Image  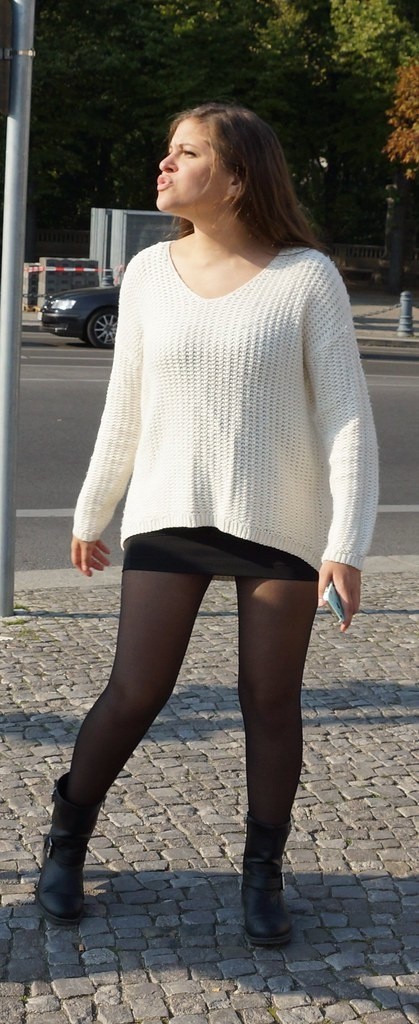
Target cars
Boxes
[37,284,120,350]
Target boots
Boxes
[35,772,107,927]
[240,811,292,944]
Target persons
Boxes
[34,105,380,947]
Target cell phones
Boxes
[323,581,345,623]
